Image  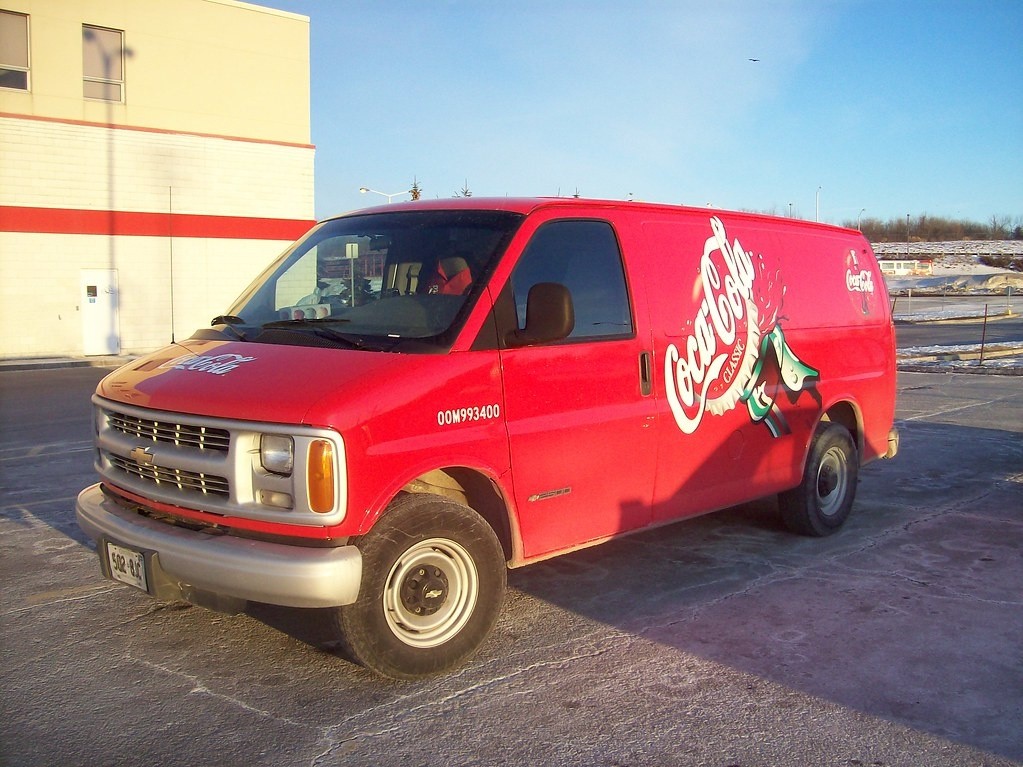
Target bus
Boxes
[876,259,934,276]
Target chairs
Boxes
[423,255,471,295]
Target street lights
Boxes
[815,185,823,222]
[906,213,911,242]
[856,208,867,230]
[788,202,793,218]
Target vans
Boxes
[74,194,900,682]
